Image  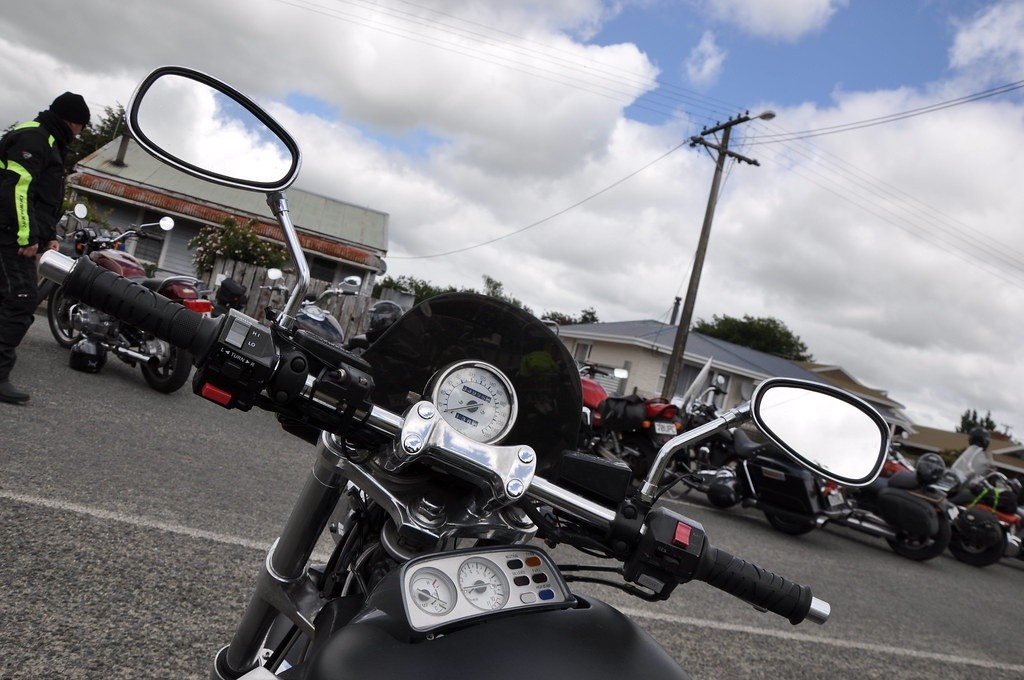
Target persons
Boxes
[0,90,91,402]
[212,277,248,318]
[952,425,995,483]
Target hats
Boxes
[50,92,90,124]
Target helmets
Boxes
[968,426,990,451]
[365,300,404,343]
[70,339,107,373]
[916,453,946,484]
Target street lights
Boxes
[658,109,780,401]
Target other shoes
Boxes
[0,381,30,401]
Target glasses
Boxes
[81,123,85,131]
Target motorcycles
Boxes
[40,59,890,679]
[45,199,1024,575]
